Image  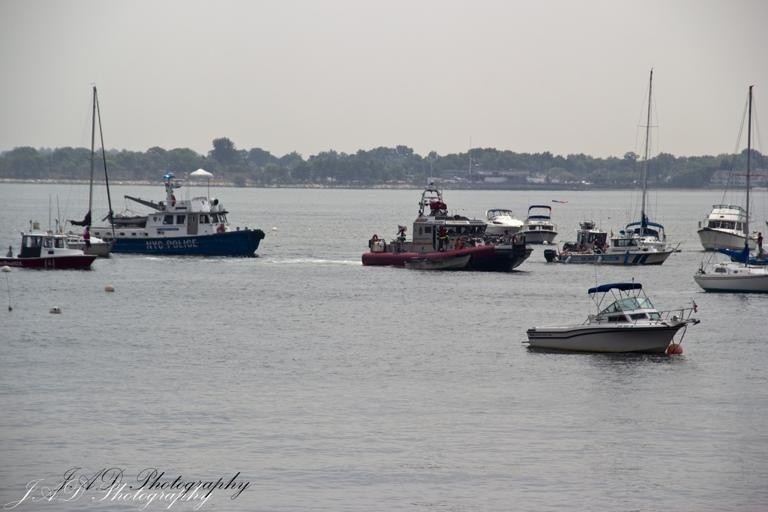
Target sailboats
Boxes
[691,82,768,295]
[626,65,671,242]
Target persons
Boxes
[498,229,512,240]
[83,227,91,249]
[756,232,763,252]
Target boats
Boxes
[0,229,100,269]
[51,83,120,257]
[524,275,706,355]
[692,202,759,251]
[90,165,271,260]
